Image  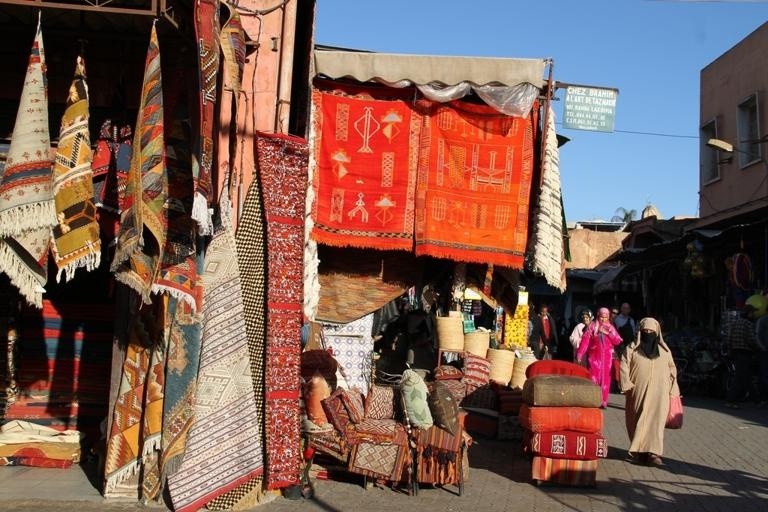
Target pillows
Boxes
[306,370,365,434]
[400,368,460,436]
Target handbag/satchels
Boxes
[663,392,683,430]
[611,358,620,382]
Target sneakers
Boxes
[626,452,667,467]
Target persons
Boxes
[618,316,681,468]
[564,302,635,409]
[534,306,559,360]
